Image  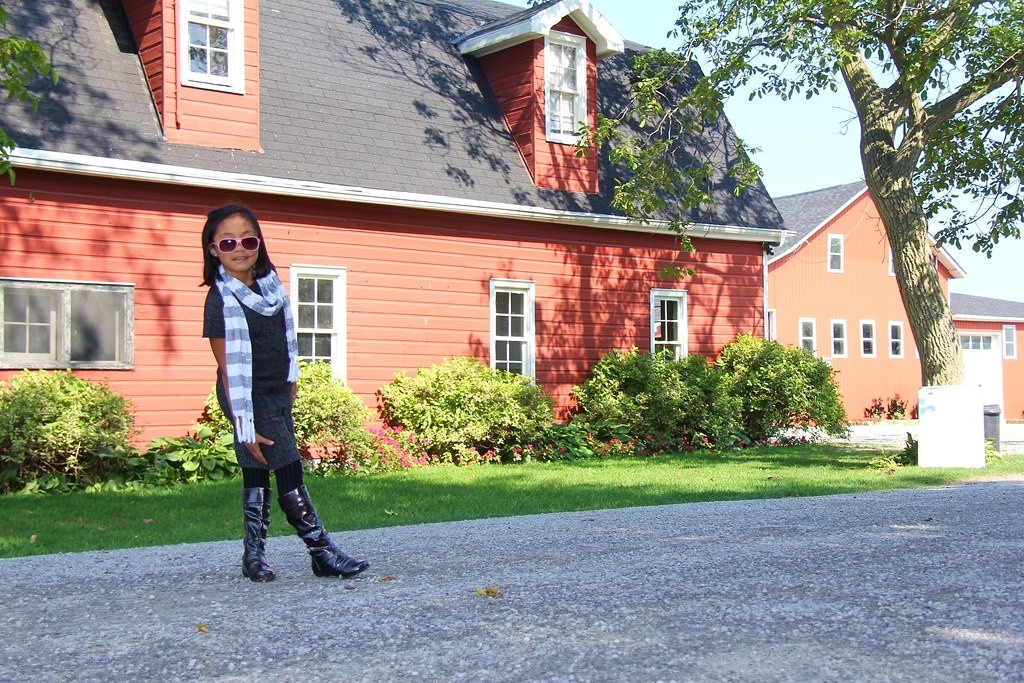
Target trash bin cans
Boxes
[983,404,1001,453]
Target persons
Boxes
[199,203,370,584]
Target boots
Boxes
[242,486,276,582]
[277,486,370,576]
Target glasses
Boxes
[211,236,261,254]
